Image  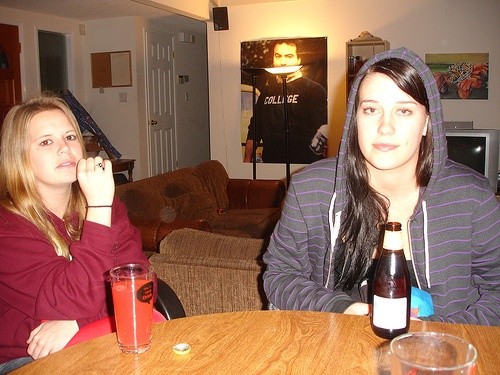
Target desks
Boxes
[3,311,500,375]
[101,158,136,183]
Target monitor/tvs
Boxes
[443,129,499,195]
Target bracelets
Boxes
[85,205,112,208]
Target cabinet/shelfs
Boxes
[346,41,389,111]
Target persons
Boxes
[243,40,327,163]
[262,46,500,325]
[0,97,168,374]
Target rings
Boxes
[95,163,105,170]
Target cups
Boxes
[109,263,153,353]
[390,332,477,375]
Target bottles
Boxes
[348,55,369,75]
[370,221,412,340]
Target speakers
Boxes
[212,7,229,31]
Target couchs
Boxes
[113,159,295,316]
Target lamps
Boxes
[243,66,303,190]
